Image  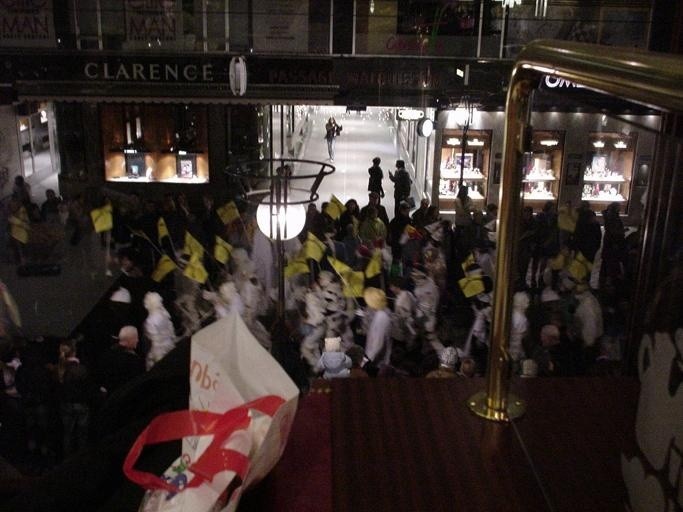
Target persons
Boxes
[324,116,341,163]
[0,156,640,512]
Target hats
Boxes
[440,346,459,364]
[363,286,388,311]
[324,336,342,352]
